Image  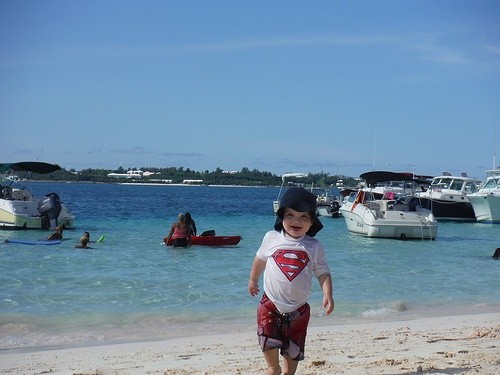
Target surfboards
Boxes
[5,238,61,245]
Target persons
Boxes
[386,192,394,204]
[48,226,63,239]
[249,187,334,375]
[167,212,196,247]
[80,232,89,247]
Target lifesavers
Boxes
[97,235,105,242]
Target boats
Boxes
[0,162,75,231]
[464,154,500,224]
[338,170,439,241]
[410,171,483,220]
[162,229,242,245]
[272,173,341,218]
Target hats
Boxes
[274,187,324,237]
